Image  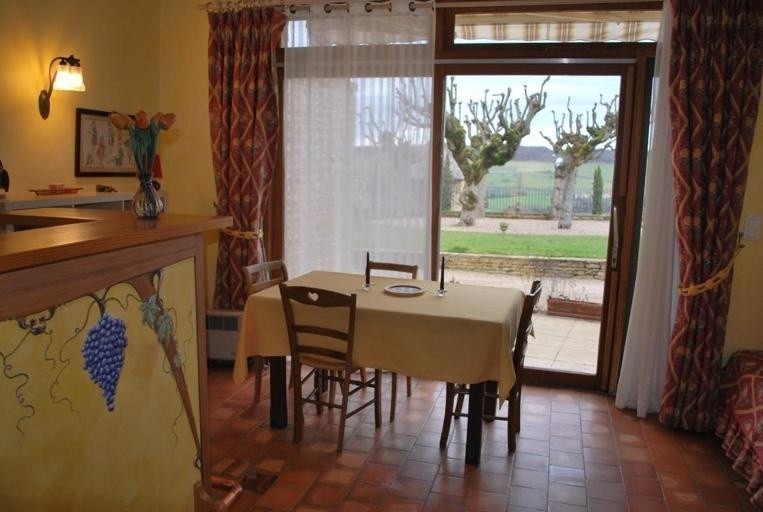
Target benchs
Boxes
[713,350,763,512]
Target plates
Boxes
[383,284,427,296]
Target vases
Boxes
[132,171,164,218]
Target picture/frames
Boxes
[74,107,142,177]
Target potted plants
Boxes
[547,294,602,321]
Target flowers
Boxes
[109,110,175,215]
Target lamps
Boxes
[38,55,86,120]
